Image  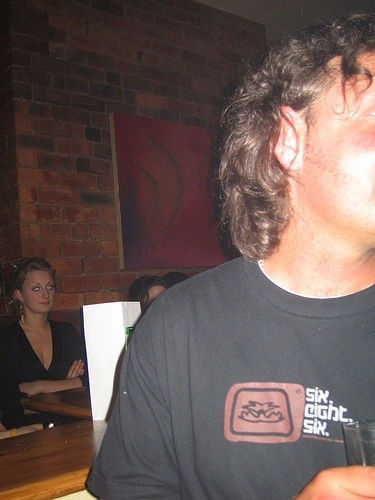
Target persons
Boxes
[128,275,170,314]
[84,12,375,500]
[0,257,89,431]
[162,272,188,288]
[0,422,43,440]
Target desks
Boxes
[0,420,95,500]
[20,387,92,418]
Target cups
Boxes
[341,417,375,466]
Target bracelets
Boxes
[8,429,16,437]
